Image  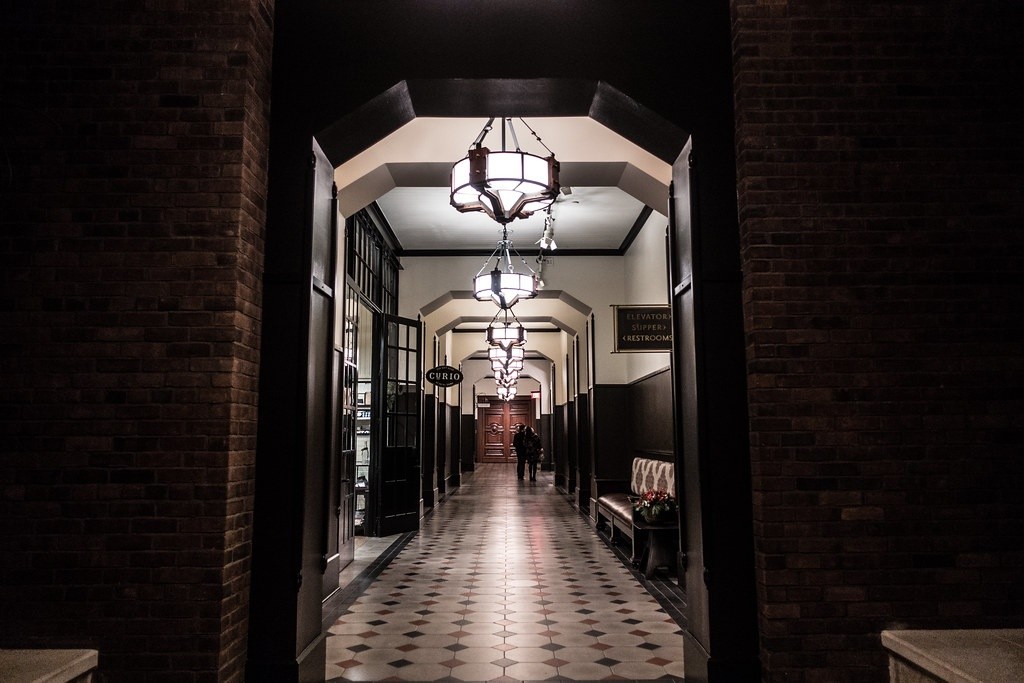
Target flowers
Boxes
[628,482,678,515]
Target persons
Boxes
[513,424,541,482]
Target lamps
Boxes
[450,84,562,223]
[472,225,538,307]
[535,214,557,250]
[485,308,529,402]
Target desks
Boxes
[881,627,1024,683]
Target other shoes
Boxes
[530,478,537,482]
[518,477,526,481]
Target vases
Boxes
[645,512,662,524]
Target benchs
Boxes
[595,449,678,569]
